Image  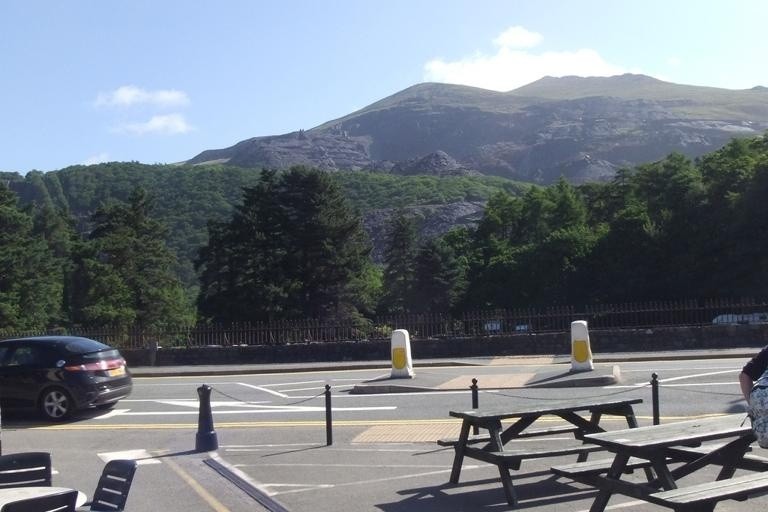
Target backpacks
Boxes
[745,368,768,450]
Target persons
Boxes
[738,344,767,407]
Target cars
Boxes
[0,336,132,422]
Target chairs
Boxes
[0,452,137,512]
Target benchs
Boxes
[437,425,768,512]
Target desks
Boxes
[583,411,752,512]
[449,398,643,505]
